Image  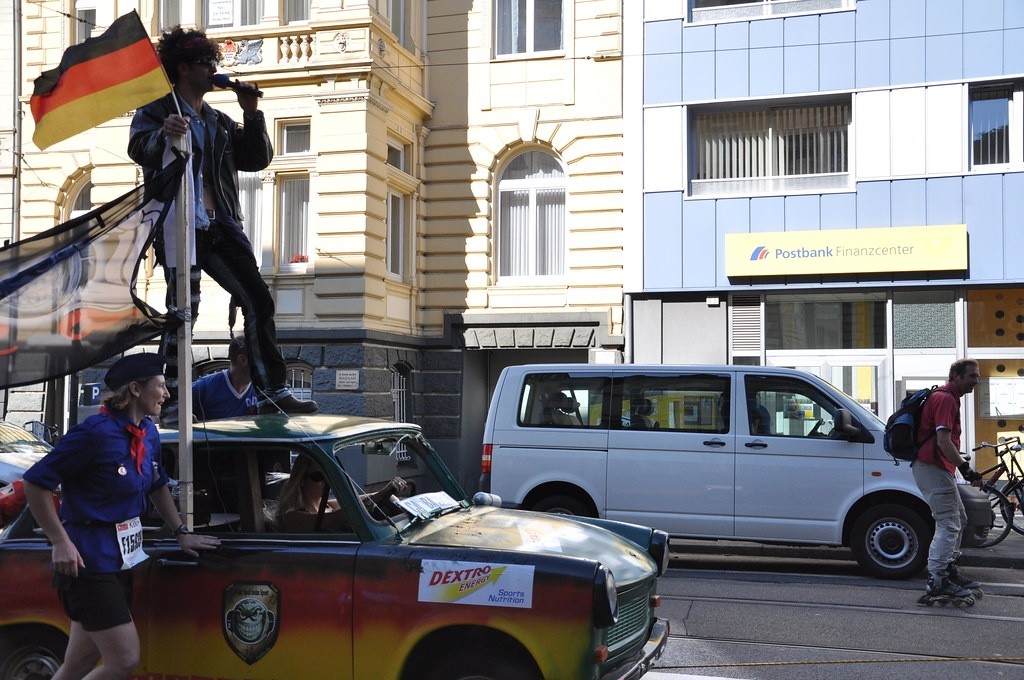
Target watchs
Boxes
[172,525,188,538]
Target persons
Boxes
[192,335,257,424]
[911,358,986,596]
[21,353,222,680]
[262,453,408,533]
[126,24,319,428]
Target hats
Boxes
[104,353,168,391]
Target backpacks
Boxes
[882,385,957,462]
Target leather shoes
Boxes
[158,410,198,428]
[258,393,319,415]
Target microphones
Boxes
[212,74,263,98]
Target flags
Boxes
[29,11,172,152]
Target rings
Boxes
[396,482,401,485]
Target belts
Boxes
[204,208,218,220]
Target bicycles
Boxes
[955,434,1024,549]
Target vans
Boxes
[479,363,996,582]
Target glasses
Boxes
[304,470,325,482]
[189,55,219,67]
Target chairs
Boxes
[543,392,583,426]
[752,402,771,435]
[630,398,655,429]
[172,475,213,532]
[719,391,731,434]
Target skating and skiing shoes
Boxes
[916,570,976,608]
[947,561,984,599]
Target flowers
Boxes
[289,252,307,263]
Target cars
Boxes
[1,412,672,680]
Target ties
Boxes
[99,406,148,478]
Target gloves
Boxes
[958,462,983,482]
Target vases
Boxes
[290,262,307,276]
